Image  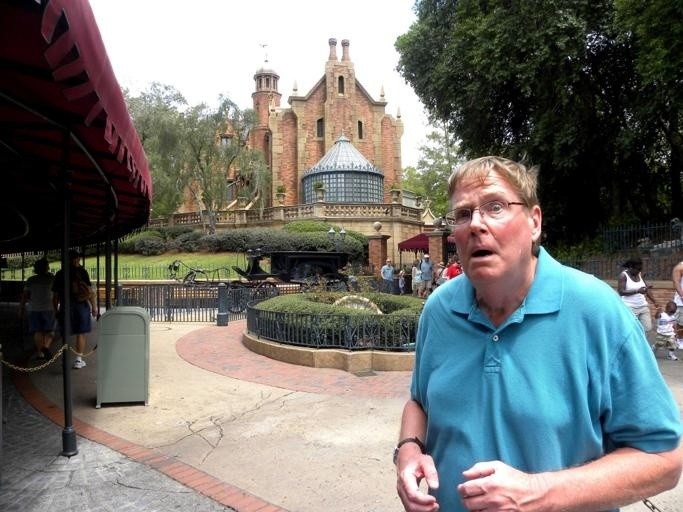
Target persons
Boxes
[672,261,683,350]
[18,259,54,360]
[618,257,660,338]
[411,253,465,299]
[393,156,683,512]
[381,258,395,293]
[397,268,406,295]
[51,249,98,369]
[651,302,679,361]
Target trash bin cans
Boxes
[399,274,413,294]
[390,278,400,294]
[95,305,150,409]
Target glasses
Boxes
[443,198,528,227]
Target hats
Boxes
[438,262,445,268]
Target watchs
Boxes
[393,436,424,464]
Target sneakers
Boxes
[73,360,86,369]
[36,347,52,361]
[667,351,679,361]
